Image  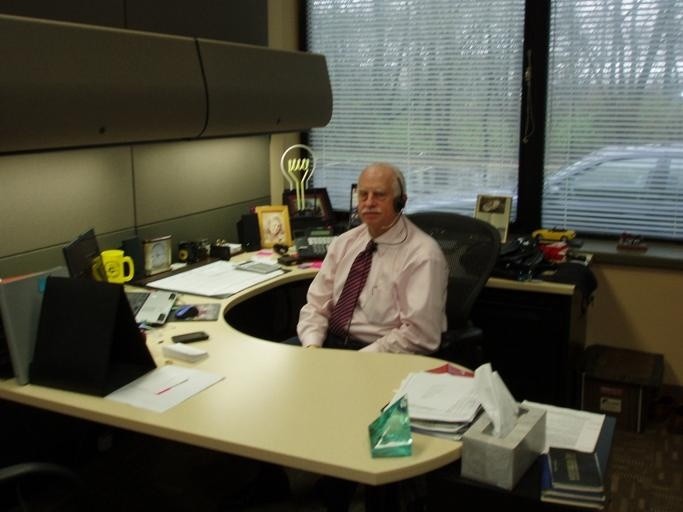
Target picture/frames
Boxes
[472,193,513,245]
[254,205,293,249]
[281,186,333,221]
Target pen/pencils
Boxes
[155,378,188,396]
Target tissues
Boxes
[461,362,545,492]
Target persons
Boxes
[282,158,448,362]
[265,215,282,240]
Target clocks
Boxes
[143,234,173,278]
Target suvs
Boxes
[407,142,682,244]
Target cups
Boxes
[101,248,134,285]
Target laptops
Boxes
[64,228,174,325]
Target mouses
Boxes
[177,307,197,320]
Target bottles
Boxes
[179,237,210,265]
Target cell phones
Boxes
[173,331,209,343]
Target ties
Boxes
[325,238,377,339]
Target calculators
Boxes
[234,261,283,274]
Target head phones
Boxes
[393,176,405,211]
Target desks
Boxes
[0,236,596,512]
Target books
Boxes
[540,447,609,512]
[236,259,280,273]
[389,369,483,441]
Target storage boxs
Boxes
[572,342,665,434]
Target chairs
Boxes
[404,211,502,367]
